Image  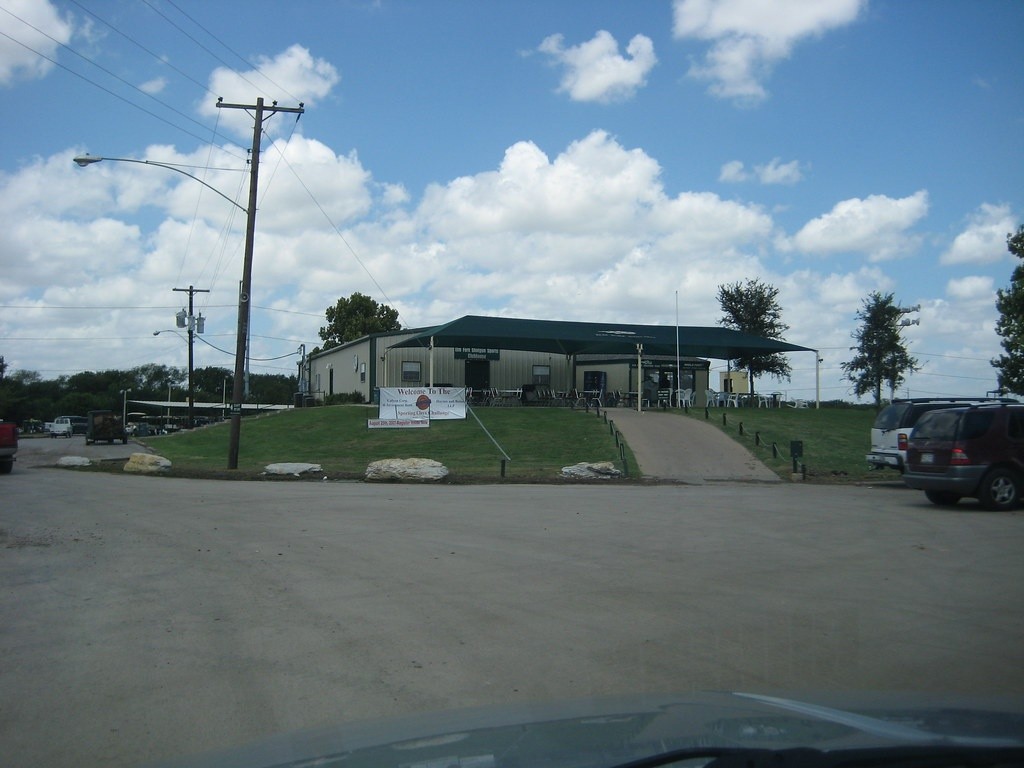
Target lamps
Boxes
[326,363,331,369]
[427,344,431,350]
[381,357,385,361]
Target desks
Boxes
[578,391,599,407]
[767,394,784,408]
[474,390,484,404]
[556,392,569,407]
[658,391,672,406]
[499,390,523,408]
[621,392,643,407]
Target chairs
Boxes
[463,385,523,407]
[535,388,651,408]
[656,388,696,408]
[787,391,808,409]
[705,388,785,408]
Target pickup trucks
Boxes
[48,418,74,438]
[43,416,87,434]
[0,421,19,473]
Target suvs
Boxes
[902,400,1024,511]
[863,394,996,489]
[86,410,127,445]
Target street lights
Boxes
[153,329,194,430]
[75,152,255,471]
[120,388,131,428]
[167,375,172,433]
[222,375,229,423]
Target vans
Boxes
[127,412,207,436]
